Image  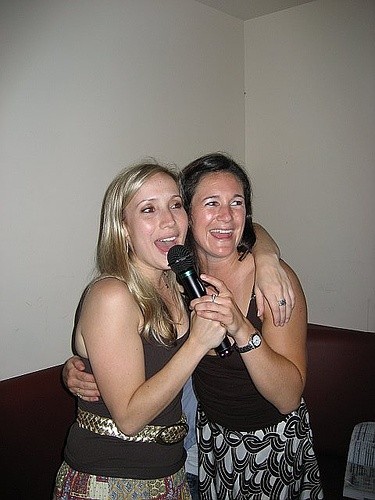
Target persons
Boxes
[61,154,327,500]
[51,164,295,500]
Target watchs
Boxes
[234,328,261,353]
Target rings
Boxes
[212,294,216,302]
[77,387,83,399]
[277,299,286,306]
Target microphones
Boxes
[167,245,233,358]
